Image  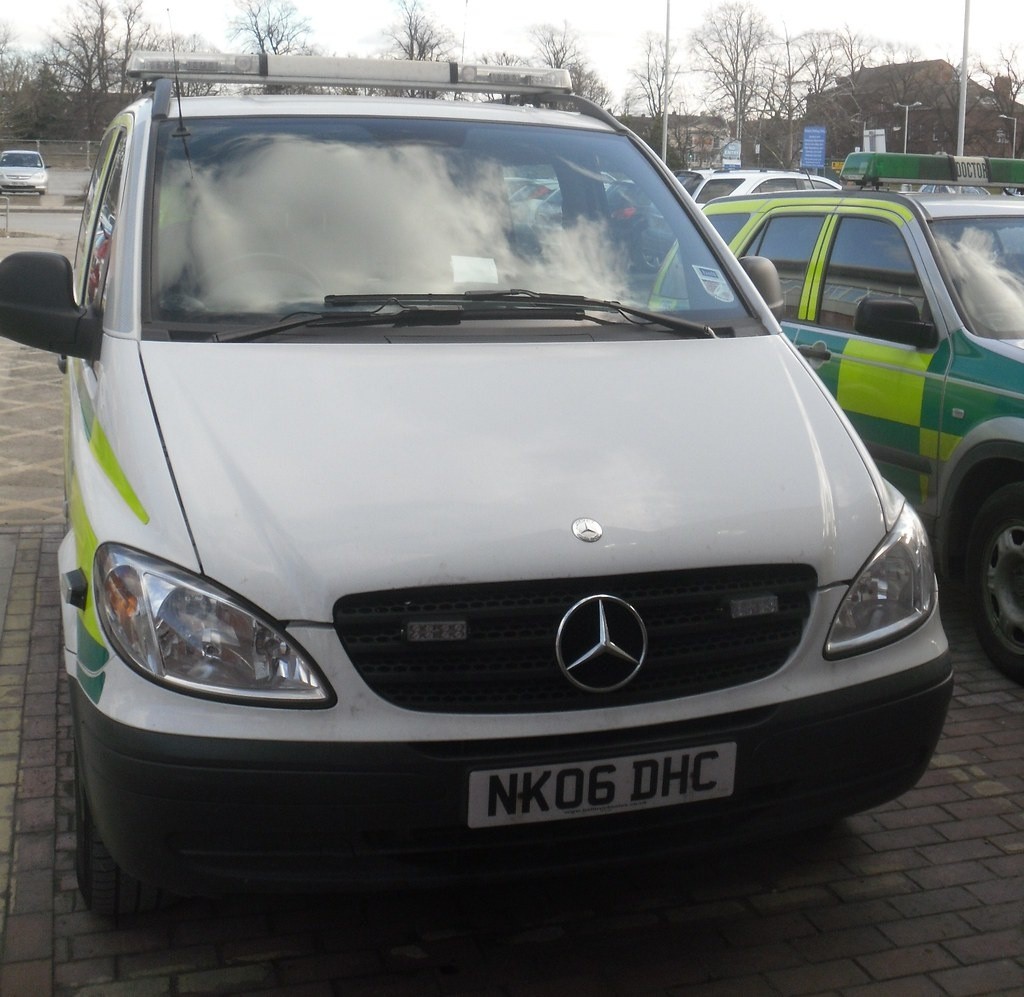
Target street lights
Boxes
[850,119,866,152]
[999,115,1017,158]
[893,102,922,154]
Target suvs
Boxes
[641,166,843,256]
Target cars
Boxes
[0,150,51,195]
[604,177,664,273]
[917,183,991,195]
[502,171,616,231]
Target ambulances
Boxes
[0,51,961,924]
[646,152,1024,686]
